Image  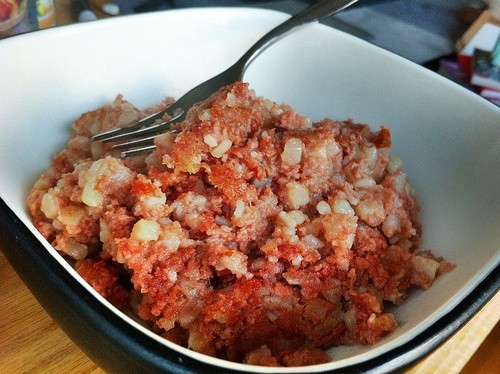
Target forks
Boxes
[90,0,359,159]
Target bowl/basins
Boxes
[0,6,500,374]
